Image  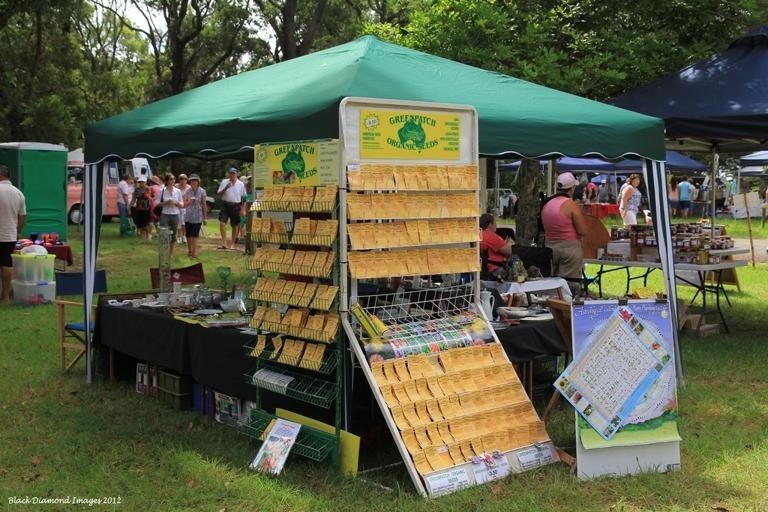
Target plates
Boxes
[194,308,224,316]
[109,302,128,307]
[500,307,529,316]
[173,312,196,317]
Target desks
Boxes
[15,242,74,272]
[93,291,375,426]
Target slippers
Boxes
[218,245,235,249]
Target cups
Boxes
[29,232,59,245]
[122,281,222,307]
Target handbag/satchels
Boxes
[153,206,162,216]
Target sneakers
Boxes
[178,236,197,259]
[121,230,152,244]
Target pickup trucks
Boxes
[65,154,152,223]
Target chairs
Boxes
[54,269,108,375]
[149,263,205,298]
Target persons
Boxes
[0,166,27,302]
[574,174,696,230]
[115,167,252,261]
[741,181,753,192]
[715,178,726,211]
[480,213,516,280]
[541,171,588,297]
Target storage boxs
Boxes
[10,279,56,306]
[10,253,56,283]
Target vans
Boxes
[107,157,153,187]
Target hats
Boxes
[189,176,200,181]
[137,175,147,182]
[557,172,580,189]
[229,168,237,174]
[150,175,160,184]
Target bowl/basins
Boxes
[219,301,240,313]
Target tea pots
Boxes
[479,288,496,323]
